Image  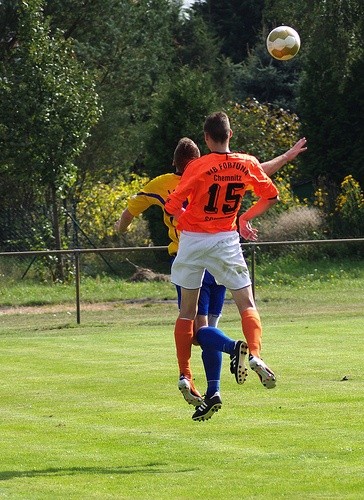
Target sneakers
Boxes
[192,391,222,422]
[248,354,277,389]
[178,374,204,407]
[230,340,249,385]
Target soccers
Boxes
[267,25,301,61]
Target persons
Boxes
[164,112,278,407]
[114,136,309,421]
